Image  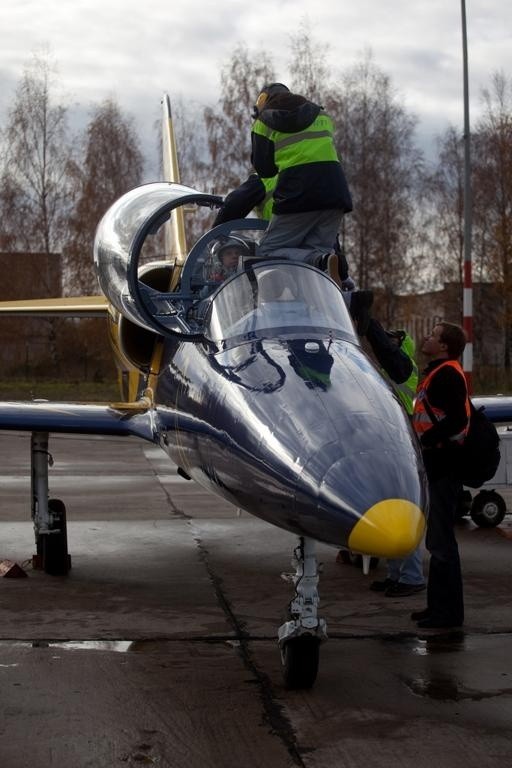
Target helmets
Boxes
[211,235,252,278]
[250,82,291,119]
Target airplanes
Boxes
[1,86,512,695]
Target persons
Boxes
[199,240,244,298]
[212,163,278,239]
[411,321,471,628]
[364,319,426,595]
[250,84,355,289]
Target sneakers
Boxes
[319,252,359,292]
[410,607,465,629]
[369,578,427,598]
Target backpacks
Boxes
[462,399,501,482]
